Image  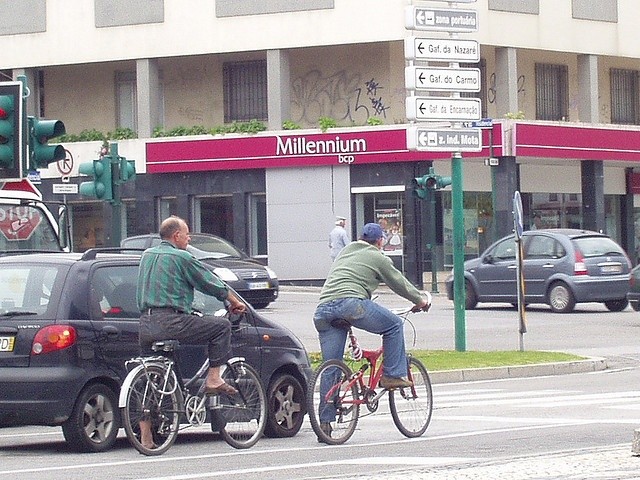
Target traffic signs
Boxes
[53,183,79,195]
[407,126,482,153]
[464,118,494,129]
[405,65,480,93]
[404,37,481,62]
[402,6,479,33]
[404,96,482,122]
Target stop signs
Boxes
[0,176,44,239]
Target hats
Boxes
[335,216,346,221]
[360,223,386,243]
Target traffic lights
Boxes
[411,177,425,201]
[26,114,66,172]
[426,179,436,192]
[0,83,23,182]
[439,176,451,189]
[77,162,105,197]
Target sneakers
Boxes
[318,422,332,442]
[380,374,414,388]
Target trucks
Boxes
[2,190,70,257]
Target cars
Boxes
[444,228,631,311]
[117,231,281,310]
[626,257,640,311]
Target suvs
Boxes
[1,242,313,451]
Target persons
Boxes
[328,216,352,261]
[531,214,541,230]
[136,215,246,456]
[312,223,432,444]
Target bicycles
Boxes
[115,284,269,455]
[311,297,433,444]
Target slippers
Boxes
[205,382,239,395]
